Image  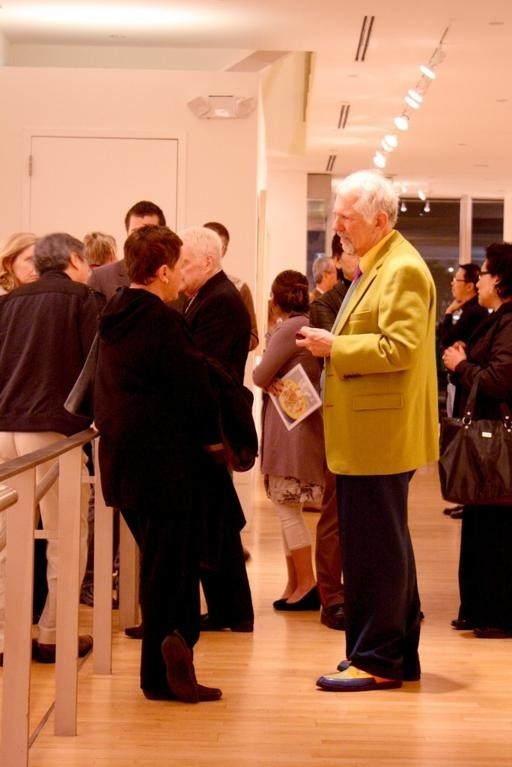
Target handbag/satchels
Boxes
[438,369,512,505]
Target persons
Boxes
[309,256,338,302]
[0,230,40,294]
[82,232,118,270]
[309,232,359,630]
[0,232,106,667]
[252,269,321,611]
[442,242,512,640]
[86,200,190,319]
[295,170,441,692]
[125,226,254,636]
[92,224,246,705]
[205,221,259,352]
[434,264,489,520]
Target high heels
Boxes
[270,584,319,612]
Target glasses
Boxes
[476,269,489,277]
[453,278,464,282]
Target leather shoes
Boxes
[320,602,346,630]
[451,619,469,628]
[143,681,222,700]
[199,613,254,633]
[162,630,199,703]
[474,625,505,638]
[451,509,463,519]
[444,505,464,515]
[39,633,94,663]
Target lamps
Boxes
[189,92,259,122]
[418,52,446,77]
[394,108,413,129]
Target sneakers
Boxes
[337,655,420,682]
[125,621,143,637]
[316,669,402,692]
